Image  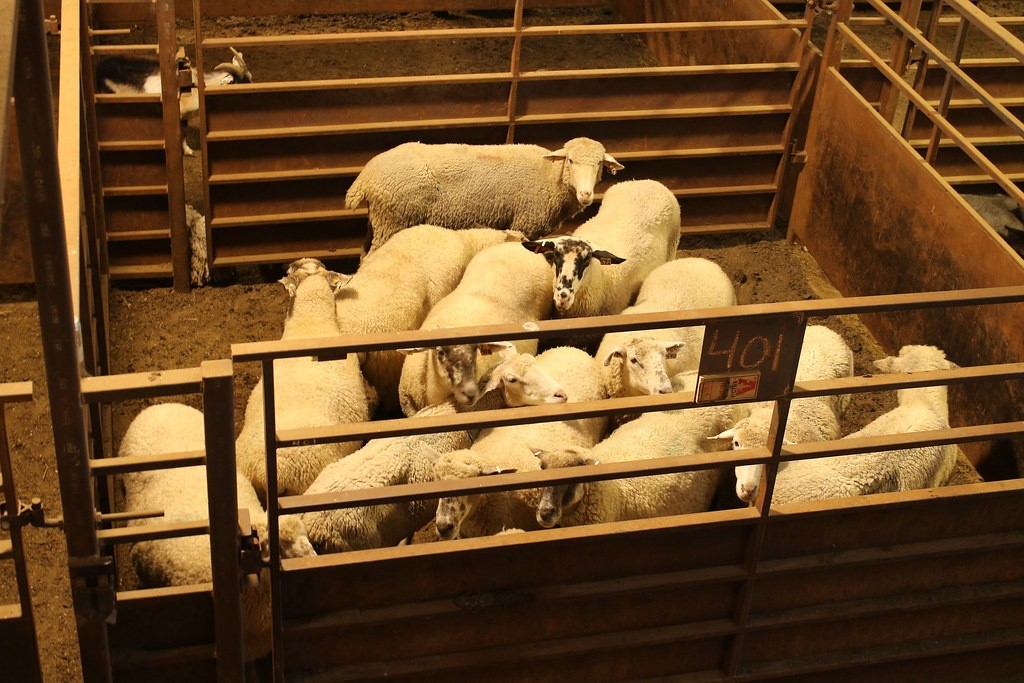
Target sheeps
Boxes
[96,46,254,157]
[117,136,964,586]
